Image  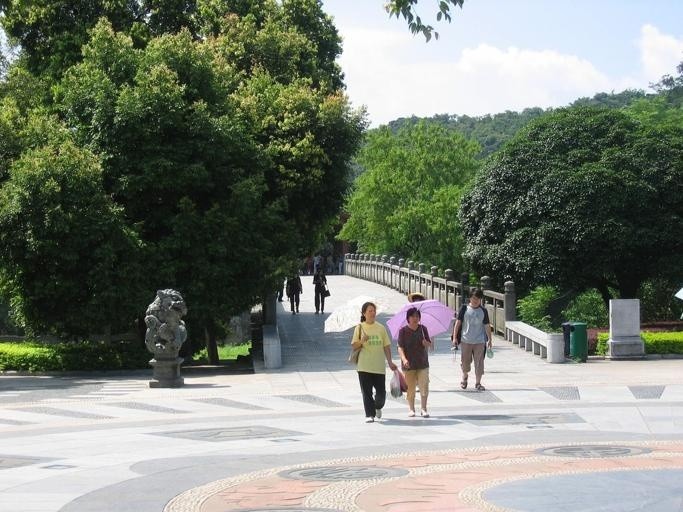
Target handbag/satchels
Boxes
[349,324,362,363]
[451,304,468,345]
[324,283,330,297]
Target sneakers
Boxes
[408,408,429,417]
[365,407,382,422]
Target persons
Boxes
[298,250,344,275]
[352,292,431,423]
[313,267,327,314]
[277,277,302,315]
[453,288,492,390]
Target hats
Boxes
[408,292,426,303]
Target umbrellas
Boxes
[324,295,457,341]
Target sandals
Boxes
[461,374,468,389]
[475,384,485,390]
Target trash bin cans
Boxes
[563,323,588,360]
